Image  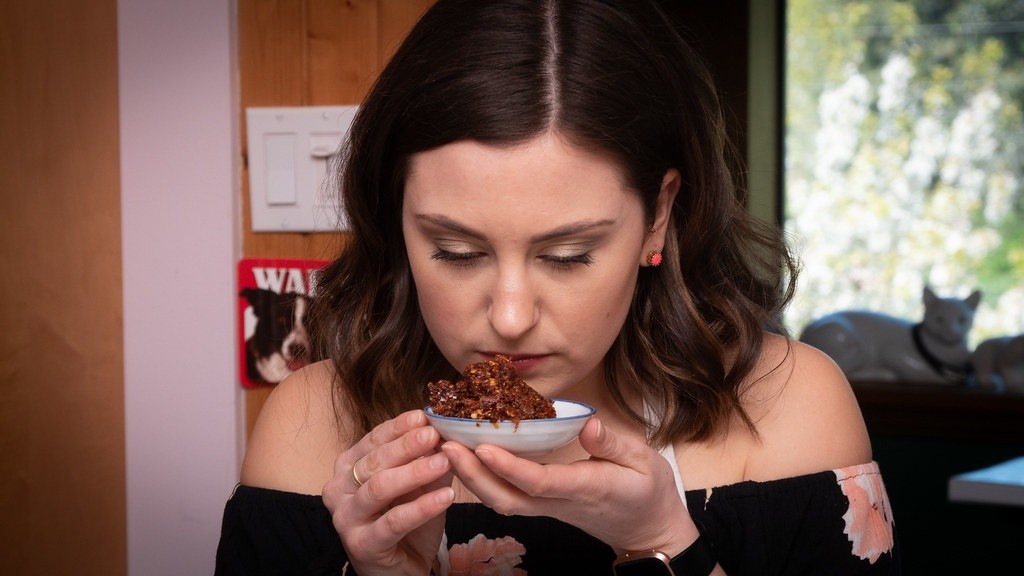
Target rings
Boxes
[351,458,363,486]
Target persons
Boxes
[214,1,908,576]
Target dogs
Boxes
[238,287,324,385]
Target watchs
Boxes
[613,517,719,576]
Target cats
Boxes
[799,286,1024,390]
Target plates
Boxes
[423,398,597,459]
